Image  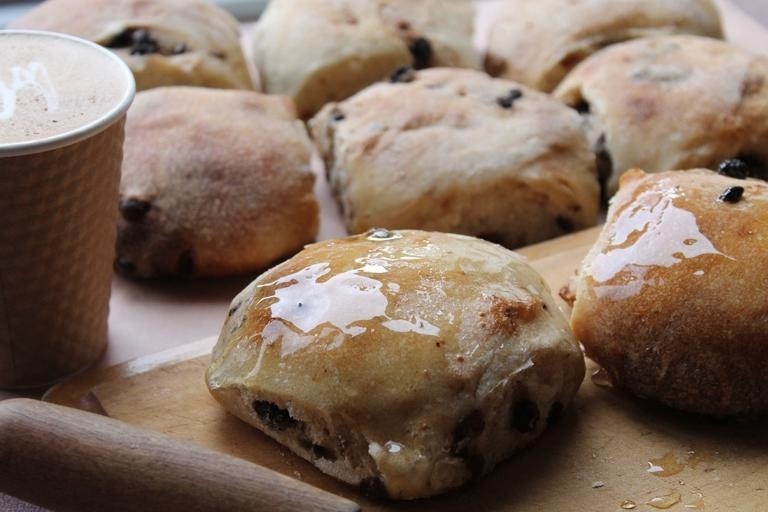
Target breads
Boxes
[113,84,321,285]
[207,227,585,502]
[251,0,768,246]
[568,166,768,419]
[6,0,256,94]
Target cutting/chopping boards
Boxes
[35,216,765,512]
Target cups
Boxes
[1,32,135,389]
